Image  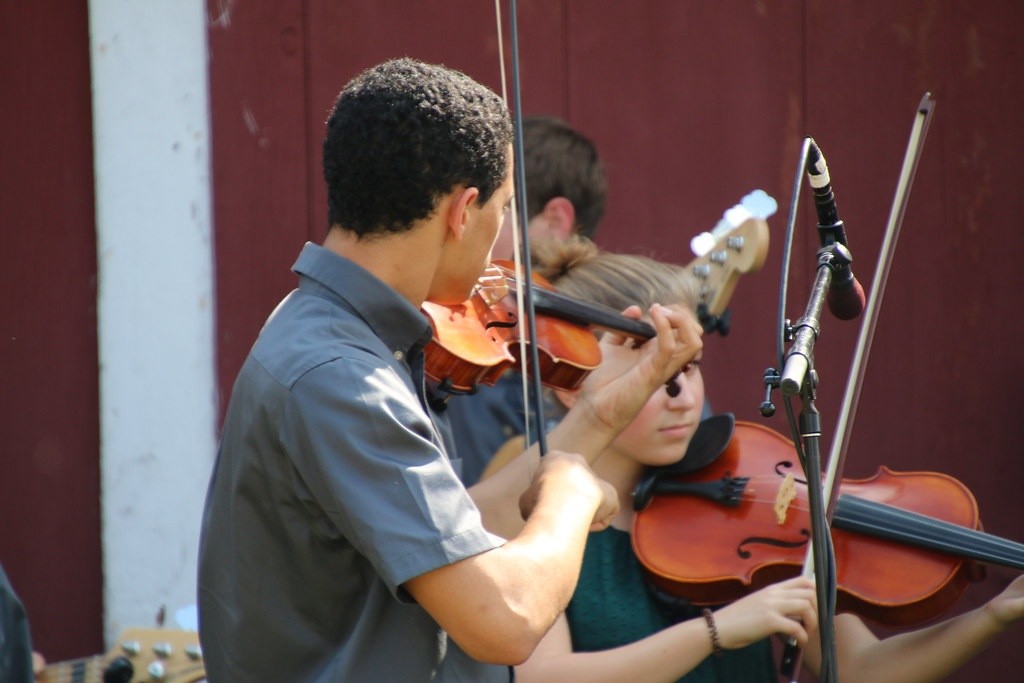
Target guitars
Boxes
[472,213,772,488]
[26,602,208,683]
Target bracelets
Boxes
[704,608,723,661]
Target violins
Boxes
[407,256,682,394]
[628,420,1024,629]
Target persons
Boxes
[197,58,1024,683]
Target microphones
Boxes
[804,134,866,320]
